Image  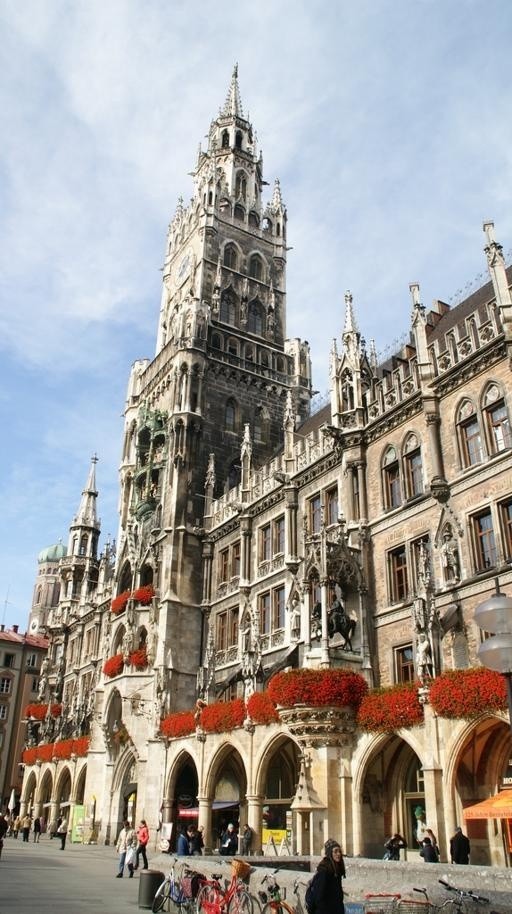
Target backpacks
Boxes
[304,872,319,914]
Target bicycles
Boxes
[412,879,498,913]
[152,849,307,913]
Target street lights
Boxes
[471,575,512,707]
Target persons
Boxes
[384,834,407,860]
[329,594,341,632]
[426,829,436,847]
[450,827,470,864]
[219,823,238,855]
[133,820,149,869]
[0,813,68,856]
[240,824,252,855]
[420,838,440,862]
[441,532,459,581]
[305,839,345,914]
[178,824,197,855]
[116,821,138,878]
[190,826,204,855]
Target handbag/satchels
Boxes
[125,846,137,865]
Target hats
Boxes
[324,839,342,860]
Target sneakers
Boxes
[129,872,134,878]
[116,873,122,877]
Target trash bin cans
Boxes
[138,870,165,909]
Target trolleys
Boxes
[344,891,435,913]
